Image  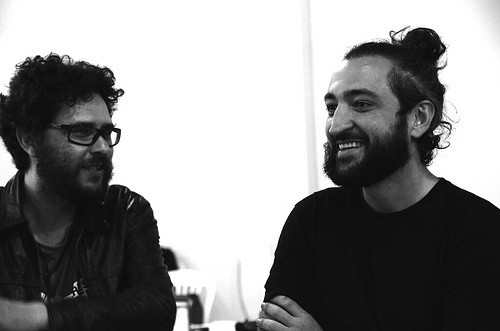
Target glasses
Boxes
[42,121,121,147]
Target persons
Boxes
[0,53,177,331]
[257,25,500,331]
